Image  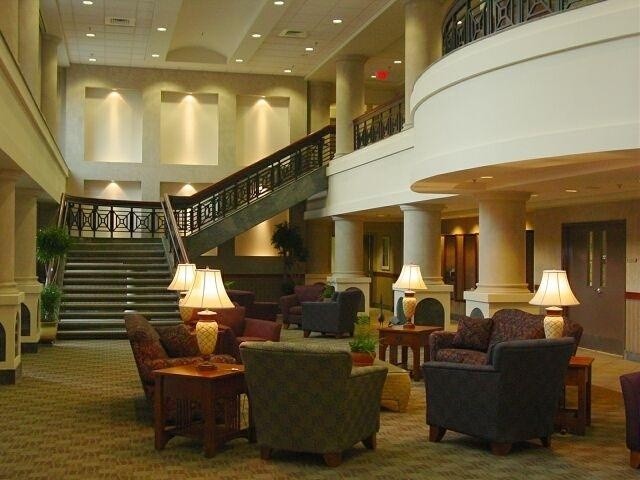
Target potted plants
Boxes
[351,337,378,367]
[39,286,61,346]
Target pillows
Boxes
[487,313,492,349]
[451,314,492,351]
[294,286,325,302]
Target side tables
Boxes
[563,353,592,439]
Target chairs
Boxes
[238,341,388,468]
[426,306,583,365]
[226,280,363,338]
[424,340,578,454]
[619,364,639,468]
[185,302,282,363]
[122,307,225,406]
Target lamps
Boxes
[393,263,428,329]
[178,269,235,370]
[528,270,582,341]
[167,262,196,292]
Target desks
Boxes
[376,325,446,382]
[151,361,242,454]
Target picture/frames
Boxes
[381,235,390,270]
